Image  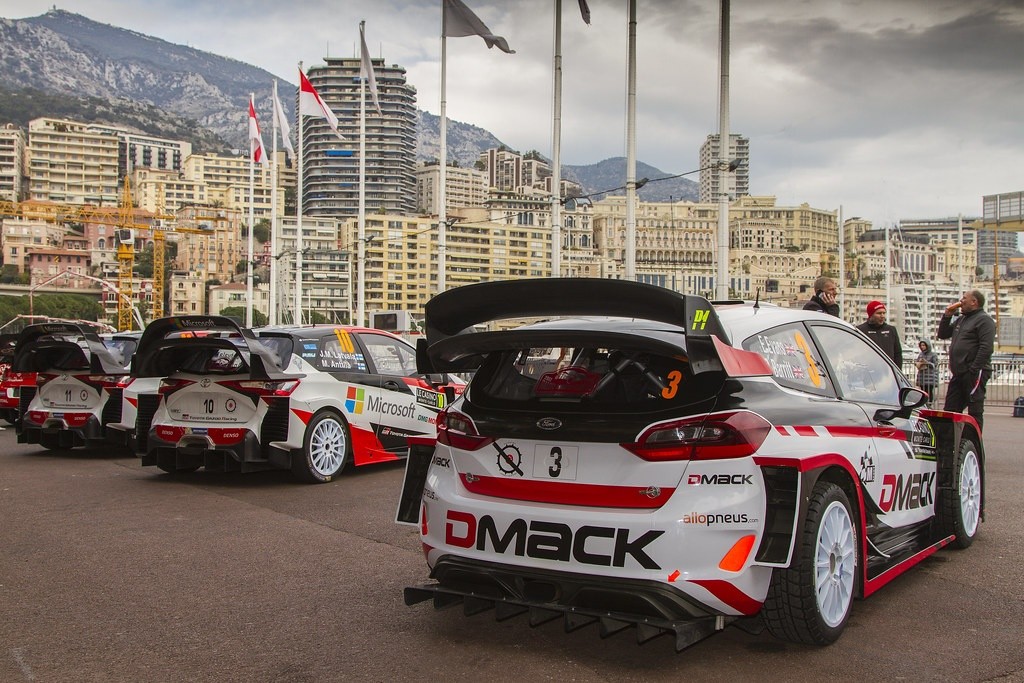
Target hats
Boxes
[866,301,886,318]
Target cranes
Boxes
[0,172,229,332]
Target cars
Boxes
[126,315,475,483]
[395,277,988,651]
[0,332,39,424]
[14,322,164,453]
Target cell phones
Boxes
[816,289,828,300]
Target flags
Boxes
[578,0,590,23]
[446,0,516,54]
[300,69,346,140]
[272,85,295,159]
[358,26,380,115]
[249,101,269,166]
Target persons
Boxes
[802,278,839,318]
[856,300,902,371]
[938,290,996,432]
[917,340,939,404]
